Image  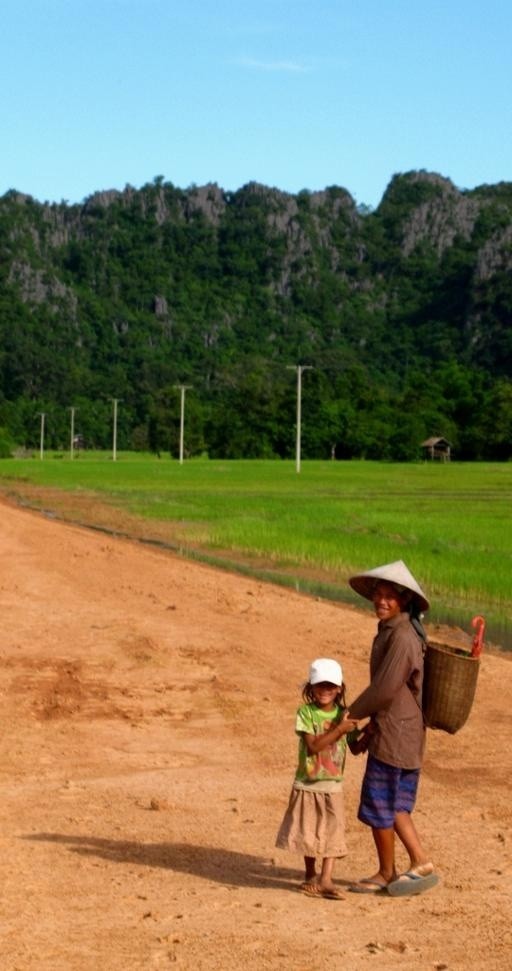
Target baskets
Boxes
[424,639,482,740]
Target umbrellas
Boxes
[467,616,487,657]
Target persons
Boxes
[329,558,440,899]
[274,658,378,902]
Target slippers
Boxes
[347,877,388,894]
[321,884,347,900]
[298,878,323,898]
[385,870,442,898]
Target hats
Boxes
[307,657,345,688]
[347,558,430,614]
